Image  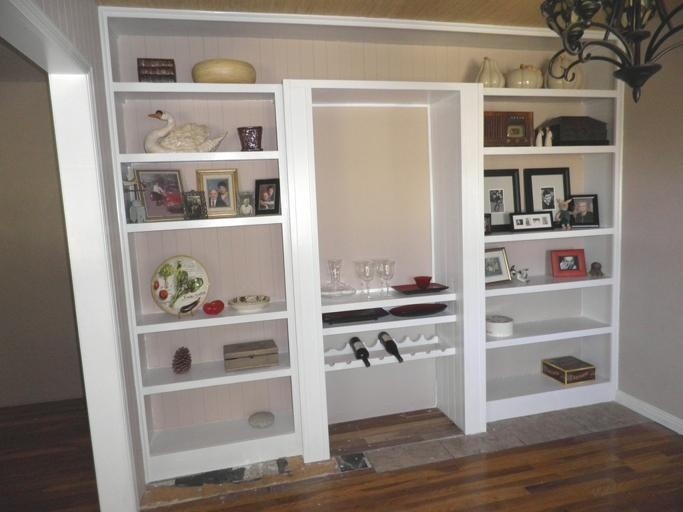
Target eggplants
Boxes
[179,297,200,313]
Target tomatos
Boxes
[154,280,158,289]
[203,300,224,316]
[159,290,168,298]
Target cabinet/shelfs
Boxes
[102,81,332,483]
[429,90,626,436]
[322,286,460,374]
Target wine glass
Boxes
[355,257,394,299]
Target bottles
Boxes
[348,336,370,369]
[320,257,354,299]
[376,331,403,363]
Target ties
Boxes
[212,199,214,207]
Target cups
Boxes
[414,276,432,289]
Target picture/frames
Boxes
[485,168,522,233]
[510,211,554,232]
[196,169,241,220]
[133,167,189,221]
[485,247,512,283]
[523,166,572,228]
[549,250,587,277]
[255,178,279,214]
[571,194,600,229]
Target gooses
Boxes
[142,109,226,154]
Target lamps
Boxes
[539,0,683,104]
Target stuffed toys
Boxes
[555,198,574,229]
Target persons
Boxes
[575,200,594,225]
[148,179,274,216]
[540,187,554,210]
[559,256,576,269]
[535,126,552,146]
[490,190,504,211]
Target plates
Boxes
[150,257,207,316]
[390,283,449,294]
[228,295,270,312]
[388,304,446,317]
[321,307,389,324]
